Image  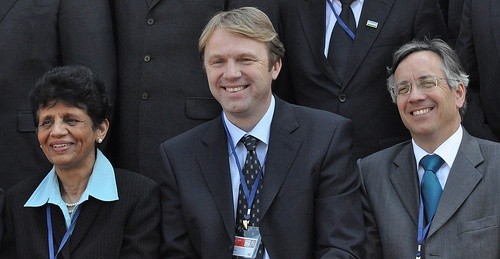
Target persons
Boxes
[157,7,363,259]
[0,0,500,193]
[357,38,500,259]
[2,64,161,259]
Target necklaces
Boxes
[65,203,77,214]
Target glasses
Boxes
[394,77,459,95]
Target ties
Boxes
[419,153,446,225]
[327,0,356,86]
[235,134,265,238]
